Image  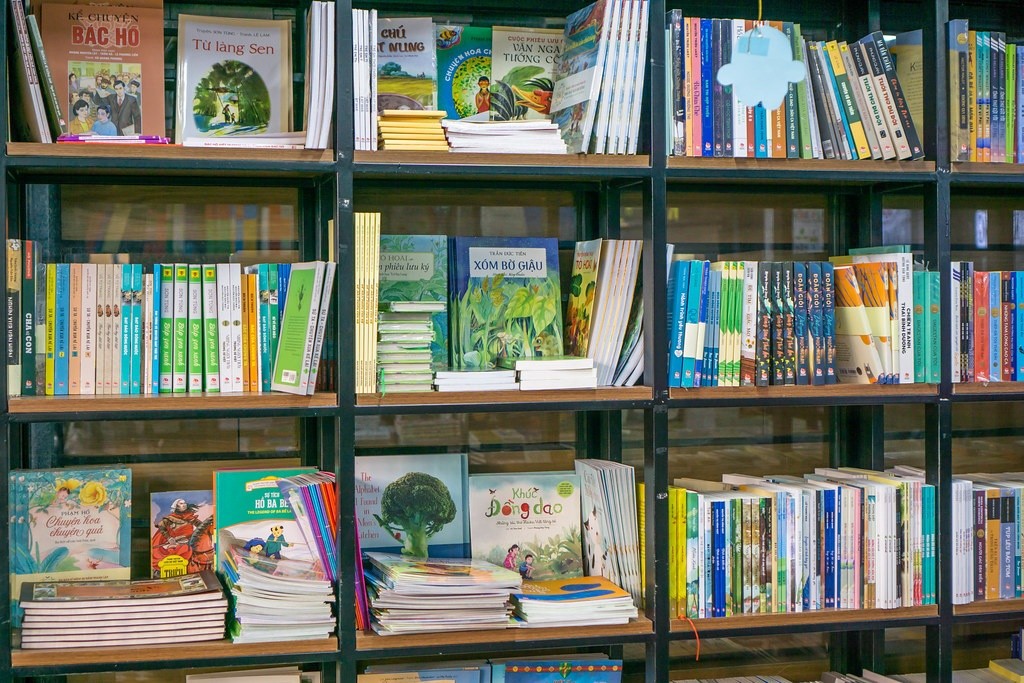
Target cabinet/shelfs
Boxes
[1,0,1024,683]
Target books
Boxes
[5,239,337,395]
[352,0,649,156]
[666,245,943,388]
[353,454,643,638]
[666,8,1024,164]
[7,0,171,145]
[328,212,675,394]
[357,653,622,683]
[637,465,1023,619]
[8,467,372,650]
[951,260,1024,383]
[176,1,335,151]
